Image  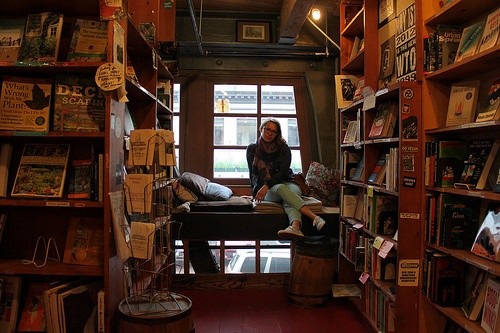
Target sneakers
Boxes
[277,225,304,242]
[313,216,326,230]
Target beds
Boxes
[172,206,341,291]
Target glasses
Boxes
[263,126,278,136]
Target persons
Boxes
[246,118,326,241]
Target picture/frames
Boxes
[235,20,273,43]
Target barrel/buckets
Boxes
[115,290,195,333]
[288,253,335,308]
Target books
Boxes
[335,0,500,333]
[0,10,176,333]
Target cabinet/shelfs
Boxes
[0,0,128,333]
[414,0,500,333]
[338,80,421,333]
[127,0,175,301]
[339,0,380,107]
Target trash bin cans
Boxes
[287,236,340,310]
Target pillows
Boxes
[171,177,198,203]
[305,160,342,208]
[178,171,234,200]
